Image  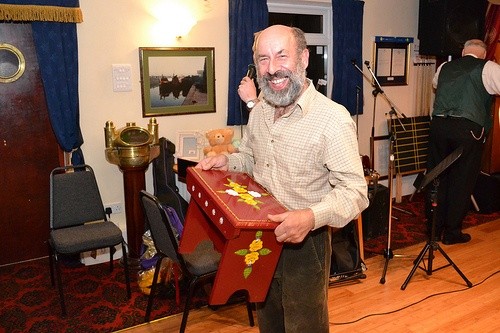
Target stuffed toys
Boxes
[203,128,239,157]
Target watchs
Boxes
[246,99,260,111]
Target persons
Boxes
[426,39,500,245]
[193,25,369,333]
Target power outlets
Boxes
[104,202,123,214]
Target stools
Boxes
[178,167,290,306]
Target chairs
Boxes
[47,164,132,319]
[139,190,255,333]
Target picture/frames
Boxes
[139,46,216,118]
[176,131,205,163]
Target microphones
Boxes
[247,63,257,79]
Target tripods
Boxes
[363,61,435,284]
[400,147,473,291]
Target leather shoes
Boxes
[442,232,471,245]
[430,233,440,242]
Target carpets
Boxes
[363,193,500,259]
[0,251,208,333]
